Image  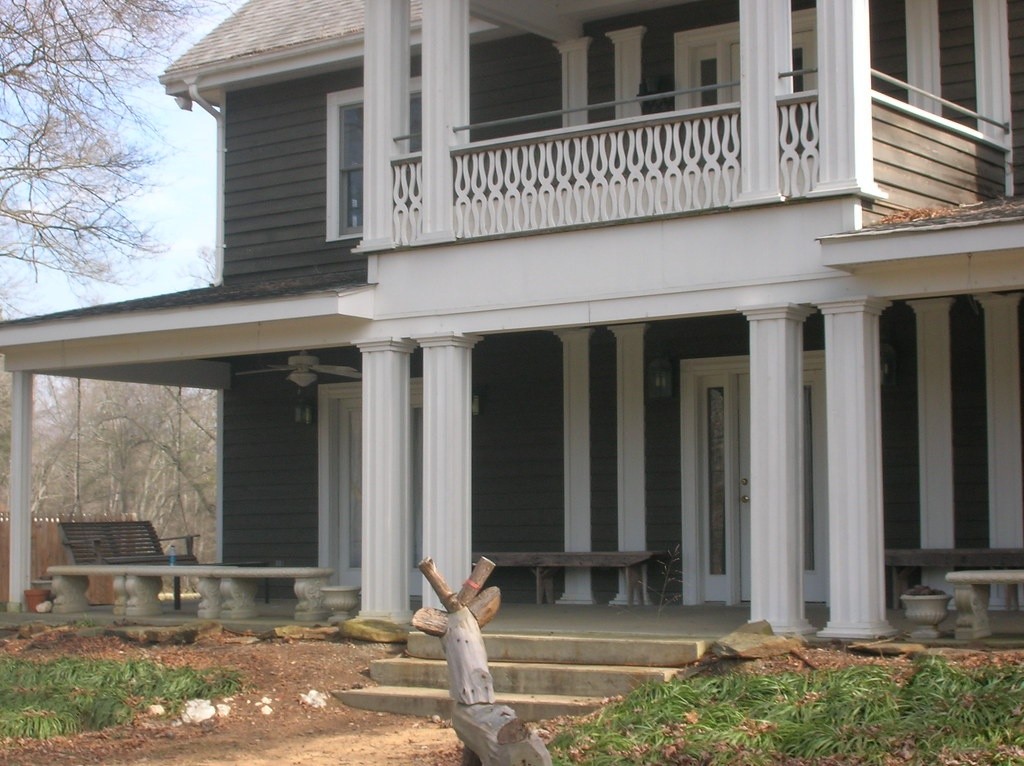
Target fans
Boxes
[235,350,362,379]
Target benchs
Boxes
[884,549,1024,610]
[58,521,202,610]
[47,565,336,621]
[471,550,660,609]
[945,569,1024,638]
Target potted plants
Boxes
[320,585,360,621]
[900,584,953,639]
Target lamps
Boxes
[880,344,898,388]
[472,392,480,416]
[636,76,660,113]
[289,369,318,388]
[296,399,313,424]
[648,357,674,392]
[175,96,192,112]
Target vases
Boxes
[24,580,53,613]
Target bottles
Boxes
[168,546,176,566]
